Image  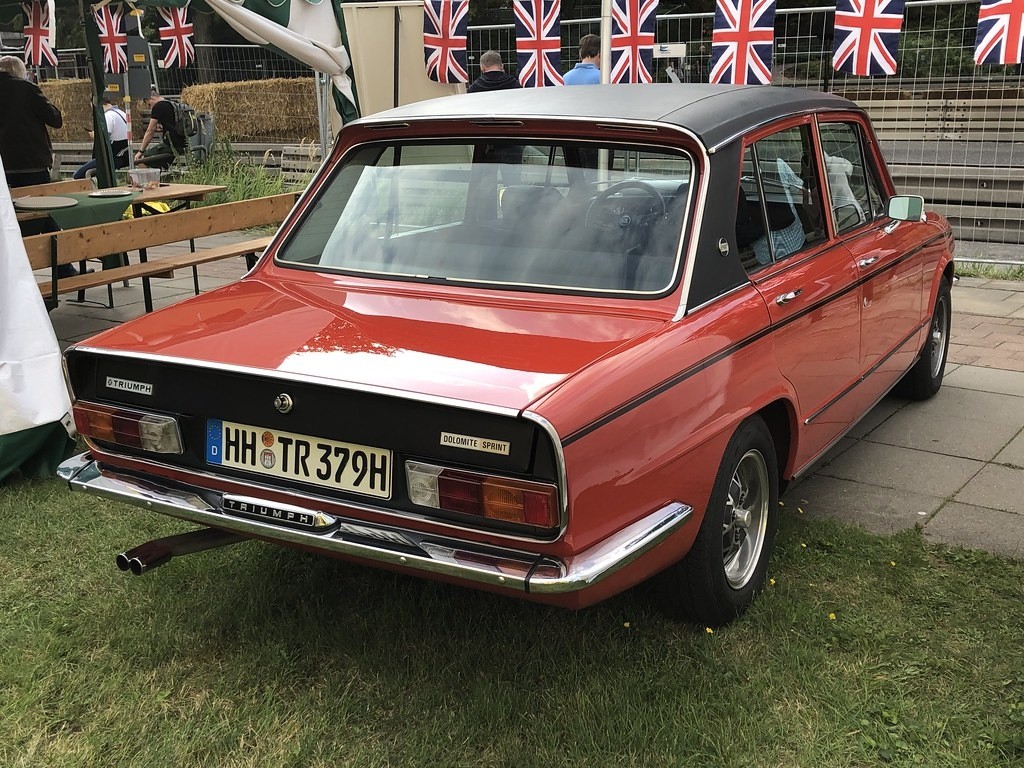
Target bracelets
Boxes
[139,149,144,153]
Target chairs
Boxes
[475,186,570,244]
[657,181,757,251]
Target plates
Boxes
[14,197,78,209]
[88,189,132,197]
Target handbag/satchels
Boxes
[111,138,130,170]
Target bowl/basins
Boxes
[128,167,162,189]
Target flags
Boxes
[831,0,906,77]
[19,0,60,68]
[973,0,1024,67]
[709,0,777,85]
[611,0,659,84]
[424,0,470,83]
[91,2,129,74]
[156,0,198,72]
[513,0,566,88]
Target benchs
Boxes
[9,178,129,287]
[23,190,305,313]
[372,241,676,291]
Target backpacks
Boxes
[160,100,194,136]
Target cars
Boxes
[56,83,957,628]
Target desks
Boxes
[15,184,227,312]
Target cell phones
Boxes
[84,127,92,132]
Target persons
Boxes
[562,34,615,190]
[73,97,129,179]
[696,46,709,83]
[134,91,188,168]
[468,50,524,218]
[0,55,95,280]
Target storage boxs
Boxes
[127,168,160,189]
